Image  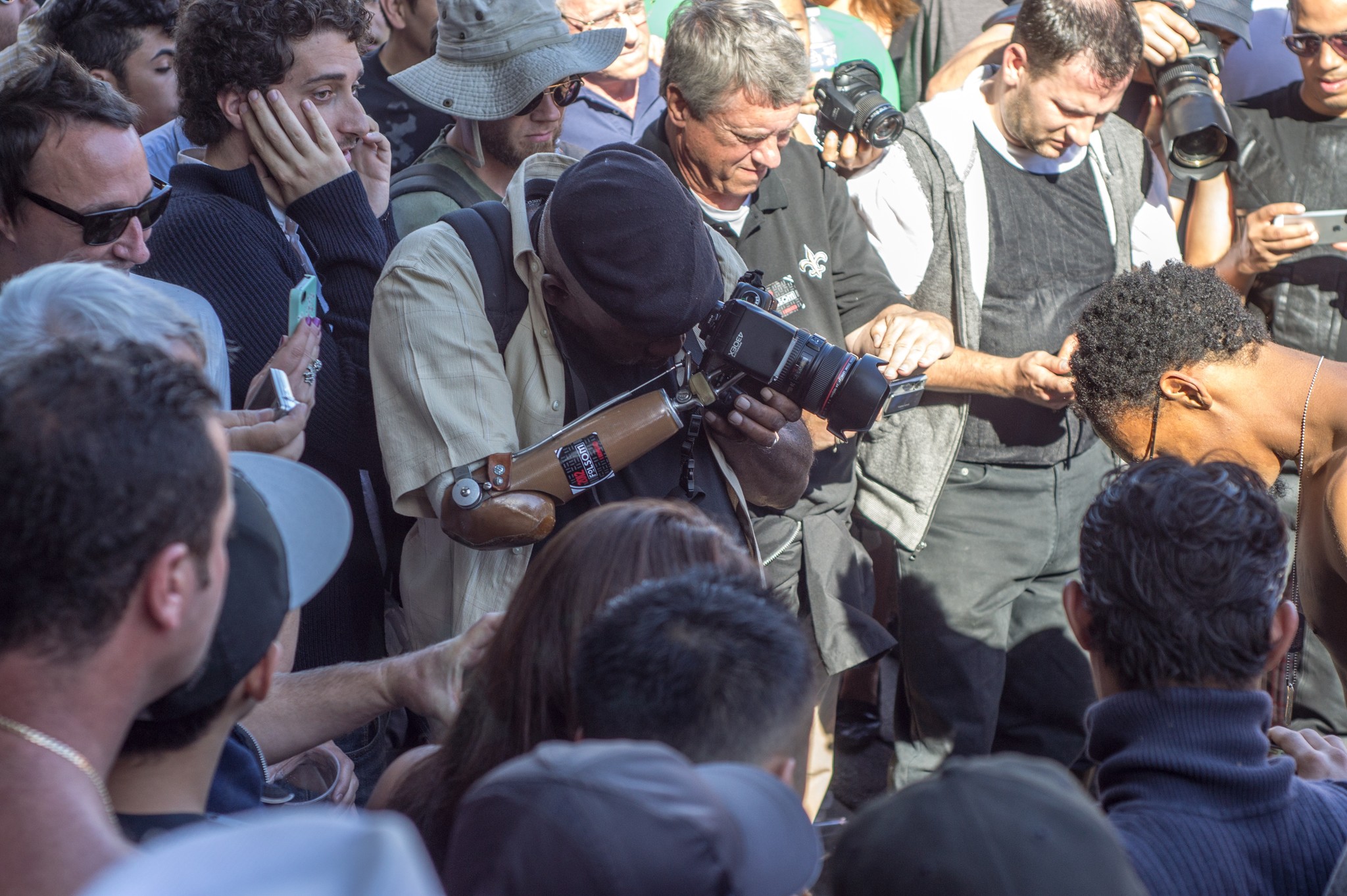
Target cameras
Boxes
[675,268,926,453]
[1146,0,1240,183]
[813,58,904,153]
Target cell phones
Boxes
[1273,209,1347,245]
[288,274,317,339]
[245,366,296,423]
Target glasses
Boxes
[561,0,644,28]
[513,74,584,116]
[23,172,174,246]
[1282,8,1347,58]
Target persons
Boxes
[368,498,756,873]
[1071,265,1347,706]
[812,758,1150,896]
[570,579,825,791]
[1184,0,1347,363]
[445,744,826,895]
[1063,453,1347,896]
[0,0,1254,808]
[0,334,235,895]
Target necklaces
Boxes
[0,717,117,825]
[1285,356,1324,727]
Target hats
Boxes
[550,143,725,338]
[1188,0,1254,50]
[387,0,627,121]
[132,451,354,722]
[445,738,822,896]
[828,755,1145,896]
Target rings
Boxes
[766,432,780,449]
[303,366,316,385]
[312,359,322,371]
